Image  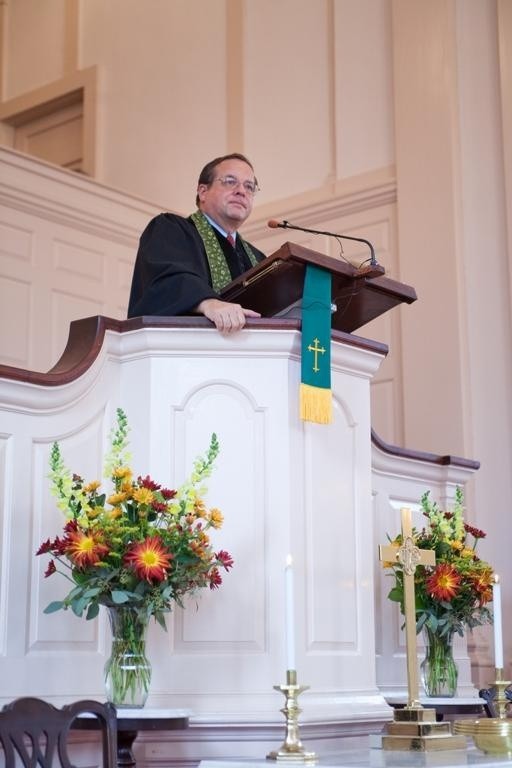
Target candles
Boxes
[285,555,297,670]
[492,575,505,669]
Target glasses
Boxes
[210,177,261,196]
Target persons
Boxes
[127,153,269,335]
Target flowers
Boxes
[386,487,499,694]
[32,407,232,705]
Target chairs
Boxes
[0,699,119,768]
[478,685,512,718]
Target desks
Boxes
[71,710,188,768]
[385,697,484,722]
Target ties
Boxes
[226,231,237,251]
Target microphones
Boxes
[267,218,376,266]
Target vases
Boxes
[420,625,458,697]
[105,608,152,709]
